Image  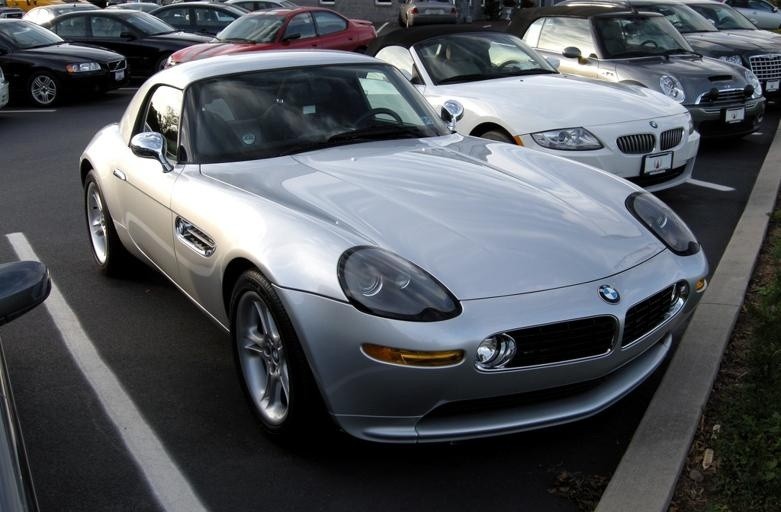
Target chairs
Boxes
[442,43,483,78]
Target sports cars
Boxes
[361,21,700,193]
[78,48,709,445]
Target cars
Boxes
[81,0,300,38]
[397,1,462,28]
[163,6,378,70]
[523,0,779,141]
[2,259,50,512]
[21,2,101,25]
[0,0,23,19]
[0,18,129,111]
[38,8,216,88]
